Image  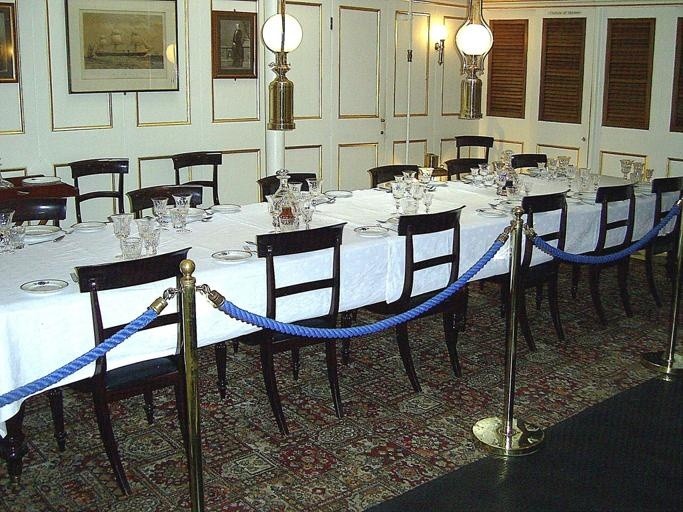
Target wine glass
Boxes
[423,188,435,213]
[419,168,434,184]
[124,237,142,259]
[632,161,645,181]
[394,175,403,181]
[111,214,132,260]
[524,182,532,196]
[581,176,589,191]
[299,191,316,230]
[413,184,425,214]
[266,195,284,233]
[390,181,403,215]
[306,178,322,207]
[401,170,415,178]
[151,197,168,232]
[288,182,303,192]
[0,208,16,254]
[506,186,519,199]
[479,164,489,188]
[571,177,580,199]
[496,162,505,170]
[591,176,599,191]
[152,230,160,254]
[646,169,654,184]
[621,159,631,181]
[471,168,478,191]
[172,194,192,233]
[405,180,414,214]
[138,218,154,259]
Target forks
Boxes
[375,223,397,232]
[69,273,78,283]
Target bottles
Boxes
[272,176,299,230]
[500,150,518,181]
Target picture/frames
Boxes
[211,10,259,79]
[0,3,20,83]
[64,0,179,93]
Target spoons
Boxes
[313,198,335,205]
[28,235,65,245]
[376,217,400,223]
[187,217,213,224]
[213,204,242,212]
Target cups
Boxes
[580,166,591,180]
[10,225,25,250]
[539,153,573,178]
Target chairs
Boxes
[455,136,493,161]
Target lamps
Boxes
[455,0,494,120]
[434,25,447,65]
[262,0,303,131]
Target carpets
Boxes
[360,370,683,512]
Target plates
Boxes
[71,221,107,232]
[500,200,521,210]
[241,243,265,261]
[354,226,388,237]
[25,225,62,236]
[20,279,68,292]
[22,177,61,185]
[172,208,204,218]
[211,249,252,261]
[325,190,353,196]
[477,208,506,218]
